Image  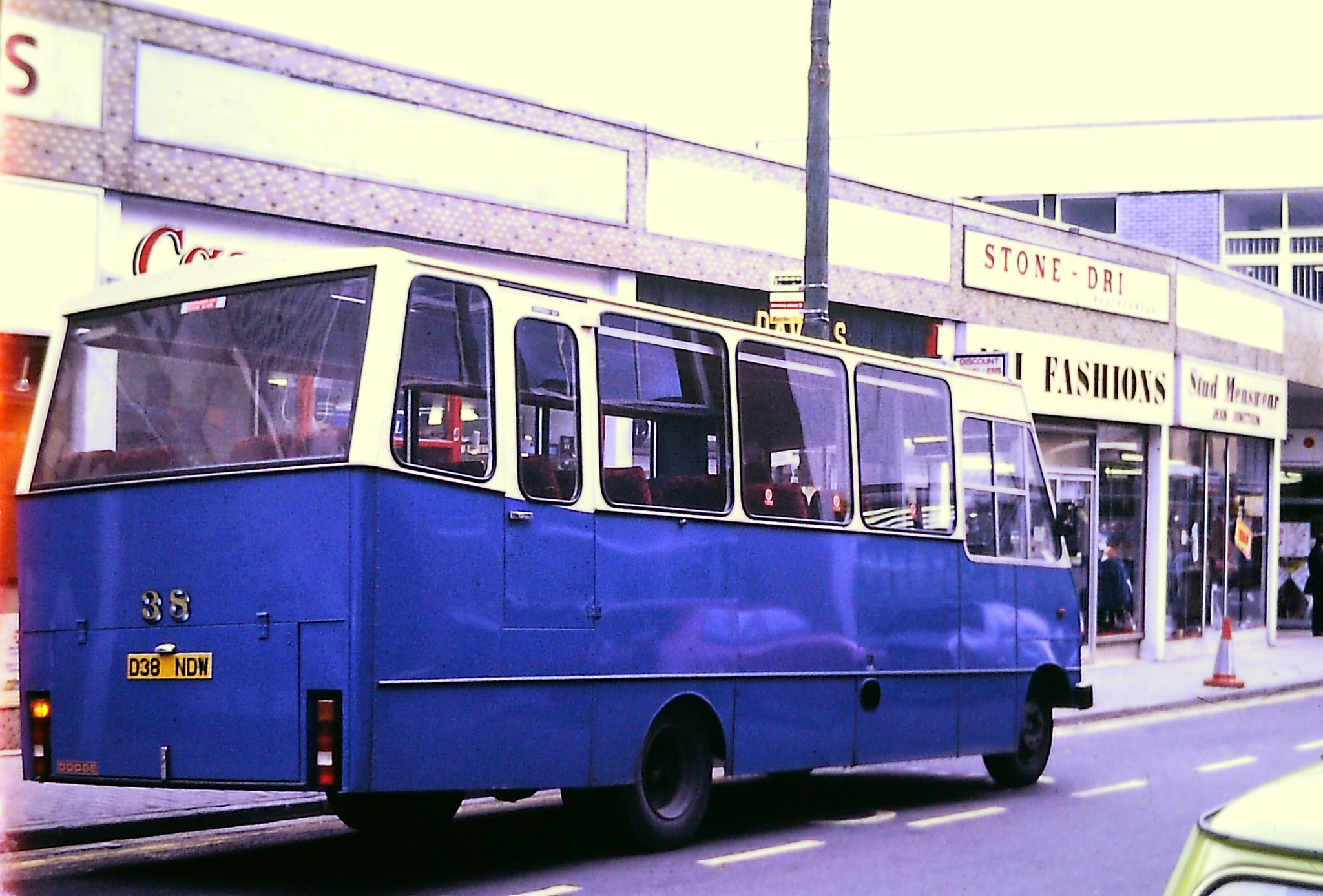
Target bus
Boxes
[14,245,1097,853]
[967,465,1222,601]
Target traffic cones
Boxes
[1203,617,1246,688]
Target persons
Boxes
[1079,545,1132,629]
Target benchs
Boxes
[523,453,561,498]
[424,458,483,475]
[607,464,657,506]
[650,473,722,510]
[744,479,809,517]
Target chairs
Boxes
[52,417,348,479]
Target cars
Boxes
[1168,763,1323,896]
